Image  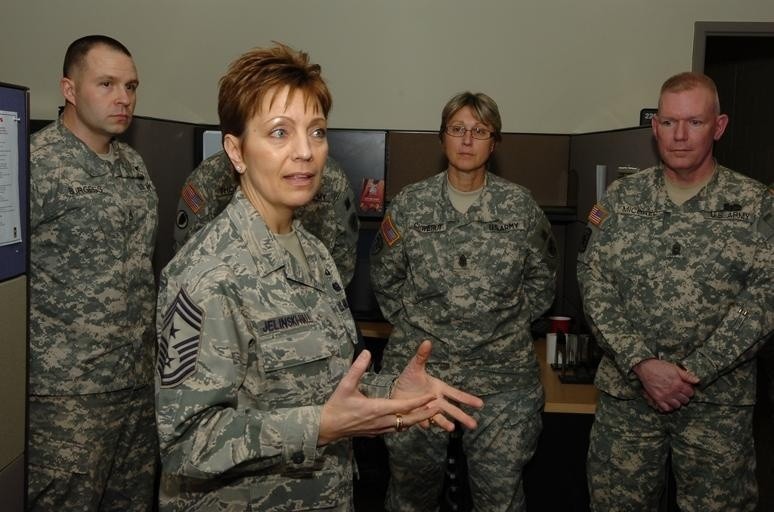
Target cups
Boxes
[551,317,571,332]
[546,333,570,367]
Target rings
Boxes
[396,414,402,433]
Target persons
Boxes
[155,42,483,510]
[175,52,361,294]
[372,93,562,510]
[574,72,774,511]
[26,35,159,512]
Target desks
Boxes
[358,320,599,511]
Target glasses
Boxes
[445,126,494,140]
